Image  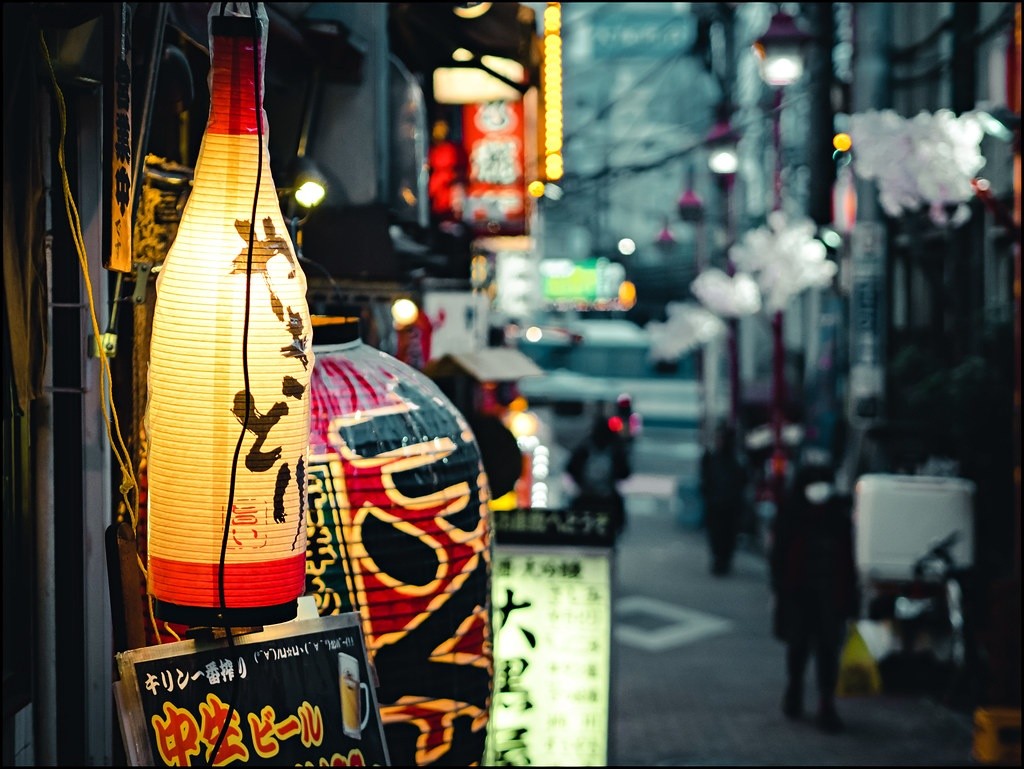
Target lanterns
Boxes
[303,315,494,769]
[147,18,306,627]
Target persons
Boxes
[769,465,862,735]
[565,397,638,544]
[698,420,747,577]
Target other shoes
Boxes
[784,688,803,718]
[816,701,837,733]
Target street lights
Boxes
[757,11,807,515]
[705,101,745,431]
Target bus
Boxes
[517,320,705,434]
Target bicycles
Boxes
[911,526,989,703]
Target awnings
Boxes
[447,348,543,382]
